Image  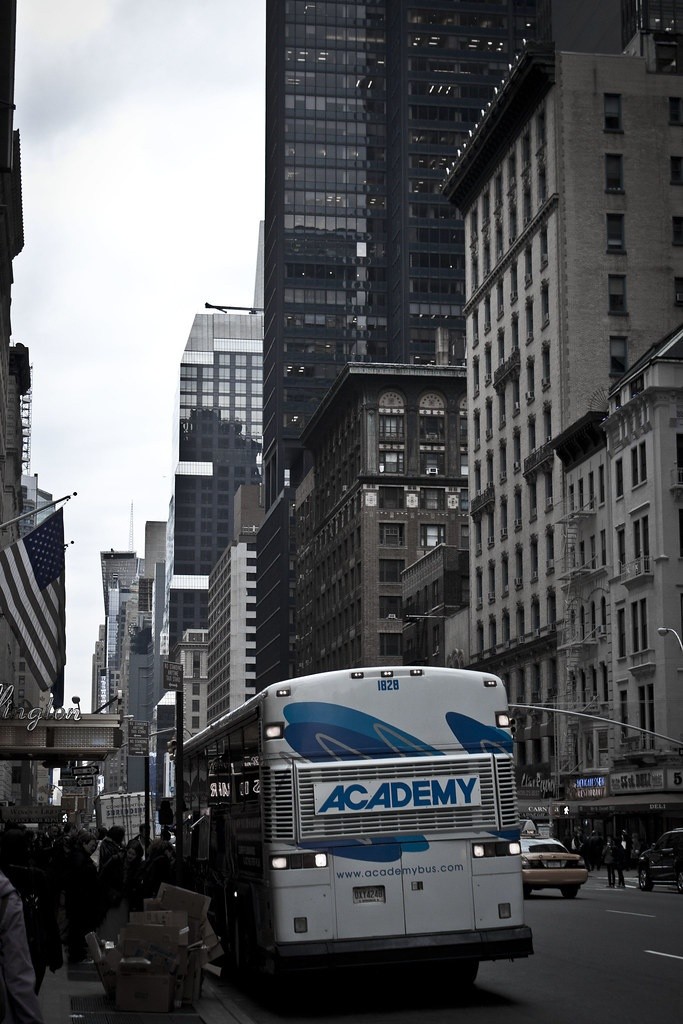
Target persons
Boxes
[563,828,648,890]
[0,824,176,1024]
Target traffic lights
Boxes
[560,805,569,816]
[509,716,522,743]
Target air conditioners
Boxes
[499,472,506,480]
[505,585,508,592]
[546,559,554,569]
[488,592,495,599]
[478,598,482,605]
[532,571,538,578]
[477,543,481,551]
[488,536,494,545]
[514,462,520,469]
[476,490,481,495]
[388,613,396,619]
[514,578,522,585]
[487,481,492,488]
[547,623,556,632]
[505,641,509,648]
[597,625,606,633]
[501,528,507,538]
[518,636,524,645]
[294,516,309,668]
[532,508,537,516]
[533,629,540,638]
[429,467,437,475]
[547,497,553,507]
[514,519,522,528]
[468,197,551,454]
[590,696,597,702]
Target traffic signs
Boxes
[71,766,99,776]
[76,777,93,787]
[128,738,148,758]
[128,720,148,739]
[161,661,183,693]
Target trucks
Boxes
[94,791,155,846]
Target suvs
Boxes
[637,827,683,894]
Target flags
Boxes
[0,507,69,694]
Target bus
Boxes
[180,665,532,1013]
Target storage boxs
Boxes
[84,882,225,1012]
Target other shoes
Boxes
[617,885,626,888]
[606,885,615,888]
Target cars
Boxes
[519,819,588,900]
